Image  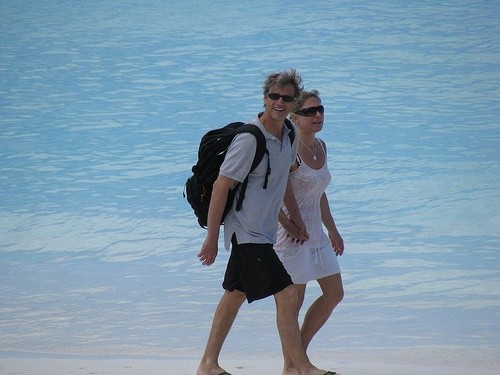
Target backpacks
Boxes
[186,116,295,229]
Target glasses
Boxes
[293,105,324,117]
[267,93,296,102]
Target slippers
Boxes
[218,371,233,375]
[323,371,336,375]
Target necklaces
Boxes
[298,138,317,161]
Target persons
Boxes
[273,89,344,375]
[196,67,342,375]
